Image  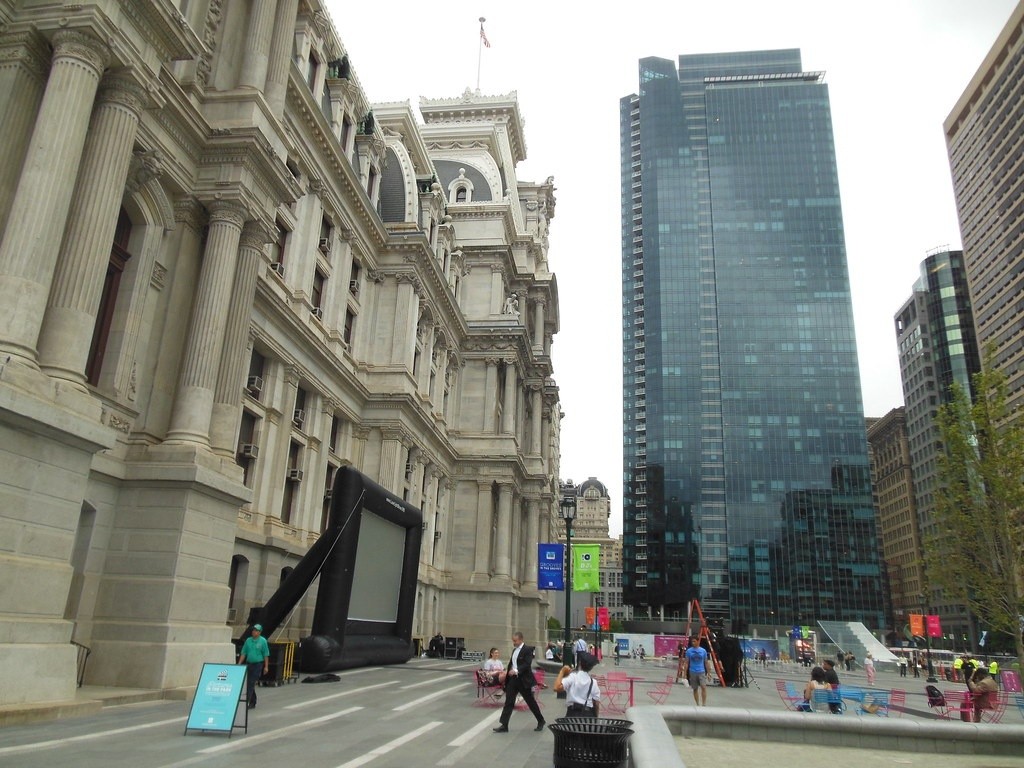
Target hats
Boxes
[251,624,262,632]
[582,652,599,667]
[824,659,836,668]
[975,668,988,677]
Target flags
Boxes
[480,25,491,48]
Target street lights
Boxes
[978,617,988,664]
[561,497,578,674]
[798,612,807,666]
[594,592,600,659]
[917,592,939,683]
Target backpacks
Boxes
[926,685,947,708]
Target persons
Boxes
[546,634,687,672]
[760,648,1020,690]
[802,667,833,714]
[553,653,602,717]
[960,668,999,724]
[503,293,520,316]
[823,660,839,709]
[493,631,546,733]
[238,624,270,710]
[484,648,507,692]
[684,636,711,706]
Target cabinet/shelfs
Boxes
[444,636,465,659]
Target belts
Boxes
[573,703,594,715]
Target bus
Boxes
[888,647,955,671]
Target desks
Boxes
[958,691,982,723]
[620,678,646,706]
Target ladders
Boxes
[676,599,729,689]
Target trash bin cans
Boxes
[546,717,635,768]
[412,638,423,658]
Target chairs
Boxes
[776,680,1024,724]
[474,669,674,711]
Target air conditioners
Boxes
[319,238,330,251]
[290,469,303,481]
[325,489,333,499]
[249,376,263,390]
[271,262,284,278]
[350,280,359,292]
[240,443,258,458]
[294,408,306,422]
[312,307,323,320]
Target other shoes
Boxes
[247,704,256,709]
[493,724,508,732]
[534,720,546,731]
